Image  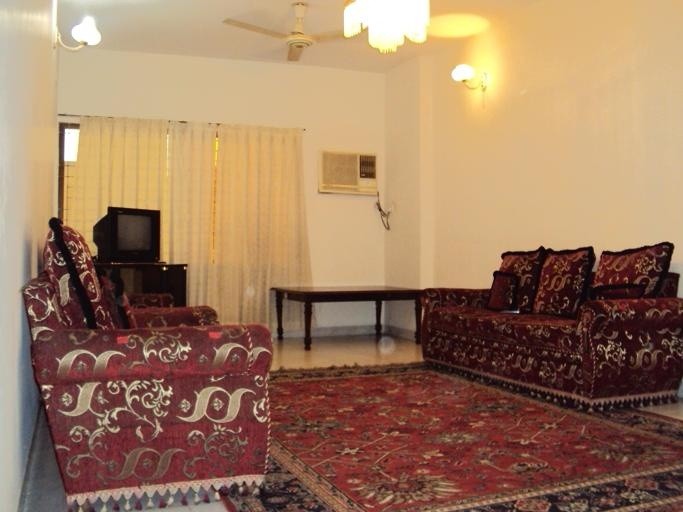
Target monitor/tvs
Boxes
[93,206,161,260]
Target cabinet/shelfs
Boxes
[94,262,188,307]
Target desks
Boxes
[271,285,424,351]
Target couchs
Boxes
[21,278,274,512]
[421,270,683,412]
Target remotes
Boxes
[501,309,521,314]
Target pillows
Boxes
[498,246,545,313]
[532,246,595,316]
[41,224,117,331]
[486,270,520,313]
[594,242,674,299]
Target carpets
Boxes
[218,361,683,512]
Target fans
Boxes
[223,3,345,61]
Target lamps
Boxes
[451,64,487,92]
[53,15,102,52]
